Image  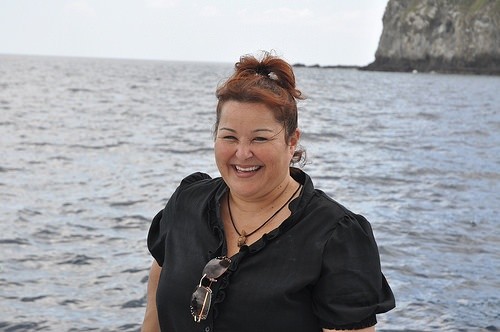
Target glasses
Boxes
[190,255,232,322]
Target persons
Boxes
[140,50,396,332]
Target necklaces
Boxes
[227,183,302,247]
